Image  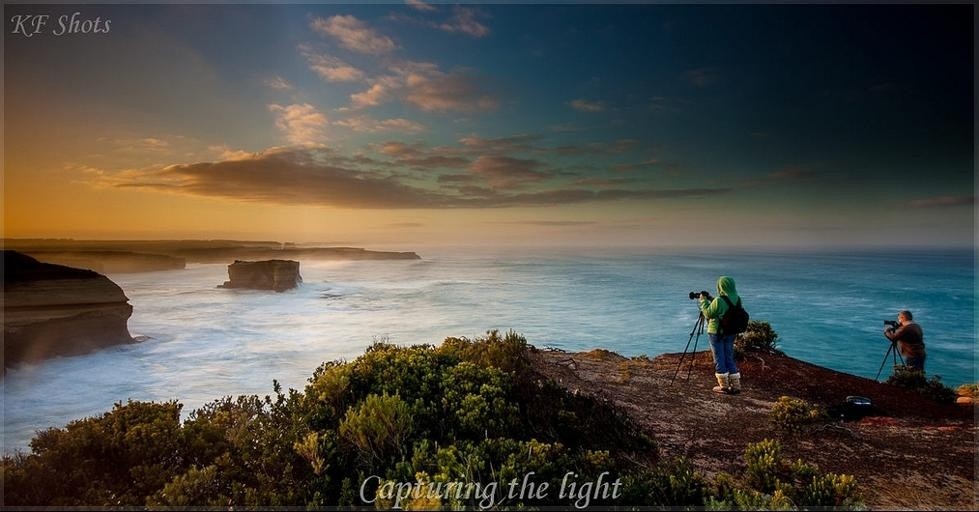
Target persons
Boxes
[699,276,743,394]
[883,310,926,376]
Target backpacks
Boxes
[718,295,749,334]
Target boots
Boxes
[728,373,742,392]
[713,372,730,393]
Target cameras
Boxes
[884,318,899,328]
[688,290,711,300]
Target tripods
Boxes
[874,342,910,382]
[669,312,712,387]
